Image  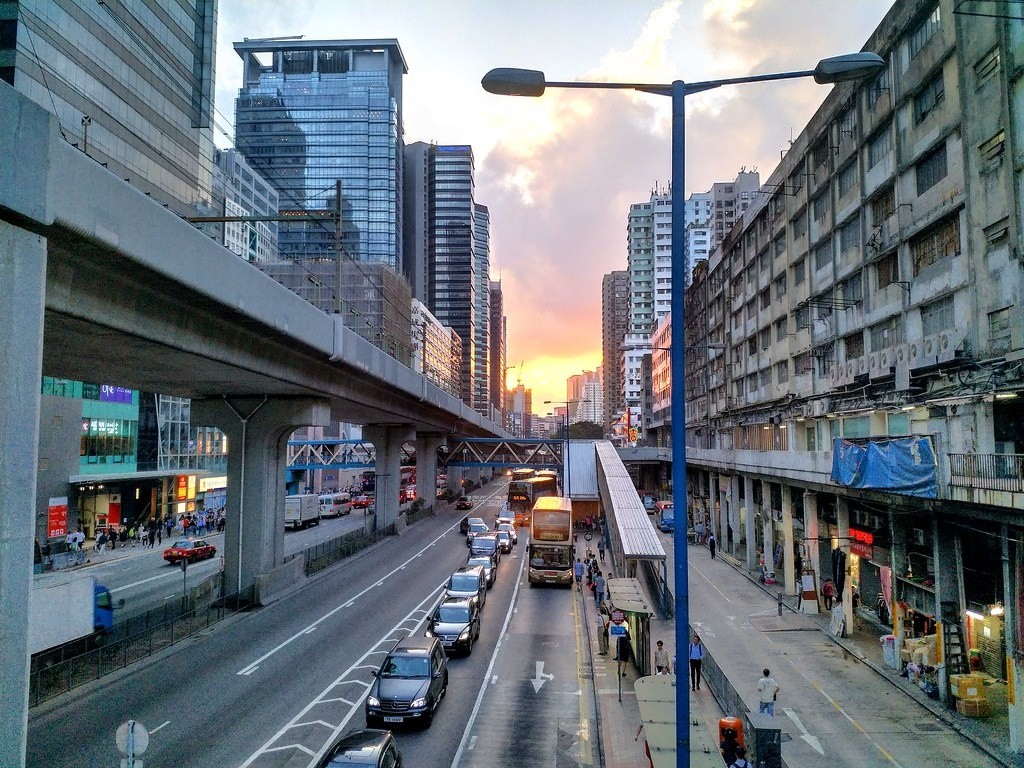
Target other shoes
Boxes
[598,652,605,655]
[604,651,608,654]
[622,673,627,676]
[692,686,696,692]
[695,684,700,690]
[613,656,618,660]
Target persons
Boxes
[757,670,780,716]
[575,538,614,610]
[822,578,834,611]
[695,521,705,544]
[634,720,654,768]
[310,481,363,497]
[574,514,605,535]
[654,640,676,676]
[533,549,546,563]
[62,517,164,557]
[689,636,705,691]
[709,532,716,559]
[34,537,51,573]
[595,602,610,656]
[720,728,752,768]
[851,587,862,619]
[760,563,768,584]
[163,503,227,540]
[614,629,631,677]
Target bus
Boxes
[512,469,535,482]
[526,496,579,589]
[506,477,556,527]
[536,470,557,497]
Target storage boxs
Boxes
[949,673,990,717]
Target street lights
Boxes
[544,399,593,498]
[478,53,887,768]
[546,413,565,497]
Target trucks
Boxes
[285,494,322,529]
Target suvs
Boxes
[364,636,449,730]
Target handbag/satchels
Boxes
[600,614,609,629]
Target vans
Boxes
[653,500,674,532]
[318,492,353,518]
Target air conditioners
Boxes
[802,397,831,418]
[830,329,957,388]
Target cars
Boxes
[353,473,474,514]
[423,594,481,657]
[163,536,217,564]
[316,729,403,768]
[637,490,660,514]
[443,502,517,612]
[456,496,474,509]
